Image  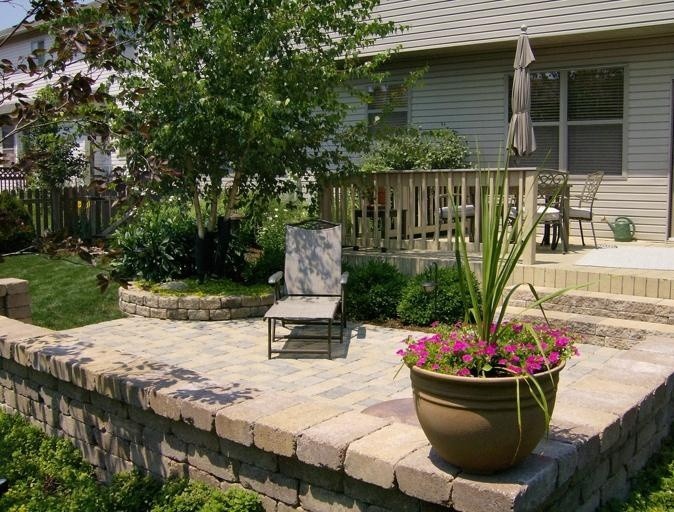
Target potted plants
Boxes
[396,136,604,478]
[360,152,393,207]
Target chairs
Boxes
[570,169,606,250]
[431,175,475,250]
[259,217,352,360]
[538,169,571,254]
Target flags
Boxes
[506,26,537,166]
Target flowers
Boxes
[394,319,580,379]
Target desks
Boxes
[353,210,407,252]
[468,182,573,250]
